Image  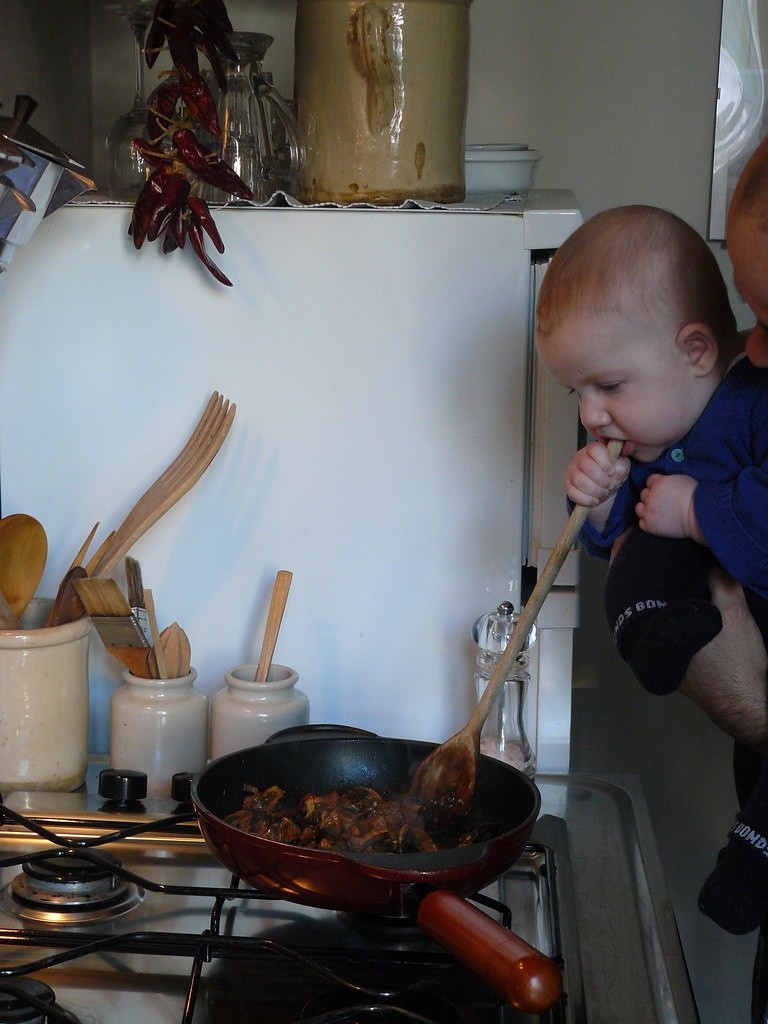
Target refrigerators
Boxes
[0,182,584,821]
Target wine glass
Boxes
[102,1,163,203]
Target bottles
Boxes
[109,665,207,794]
[471,601,537,779]
[211,663,309,759]
[199,31,307,206]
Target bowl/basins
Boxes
[466,150,542,194]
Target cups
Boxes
[293,1,471,204]
[1,596,93,794]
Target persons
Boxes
[605,136,768,1024]
[535,204,768,598]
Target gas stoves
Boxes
[1,767,703,1024]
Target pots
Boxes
[189,724,541,911]
